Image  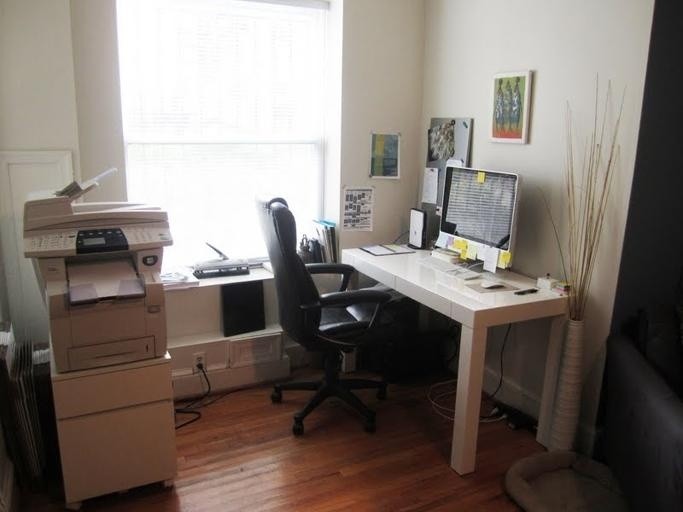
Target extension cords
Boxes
[493,401,538,431]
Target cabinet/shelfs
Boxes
[162,261,358,403]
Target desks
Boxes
[340,240,578,479]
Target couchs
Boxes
[602,322,681,512]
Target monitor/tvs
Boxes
[439,165,522,270]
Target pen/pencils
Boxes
[378,244,396,252]
[515,288,538,295]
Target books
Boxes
[312,219,339,264]
[162,271,200,288]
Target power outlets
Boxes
[193,351,207,371]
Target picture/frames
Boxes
[368,130,402,180]
[342,186,376,234]
[487,68,533,145]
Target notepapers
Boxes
[552,282,575,295]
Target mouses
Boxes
[481,282,504,289]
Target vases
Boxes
[547,316,587,465]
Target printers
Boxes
[22,166,173,374]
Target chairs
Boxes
[257,193,392,437]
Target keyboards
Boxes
[430,261,482,281]
